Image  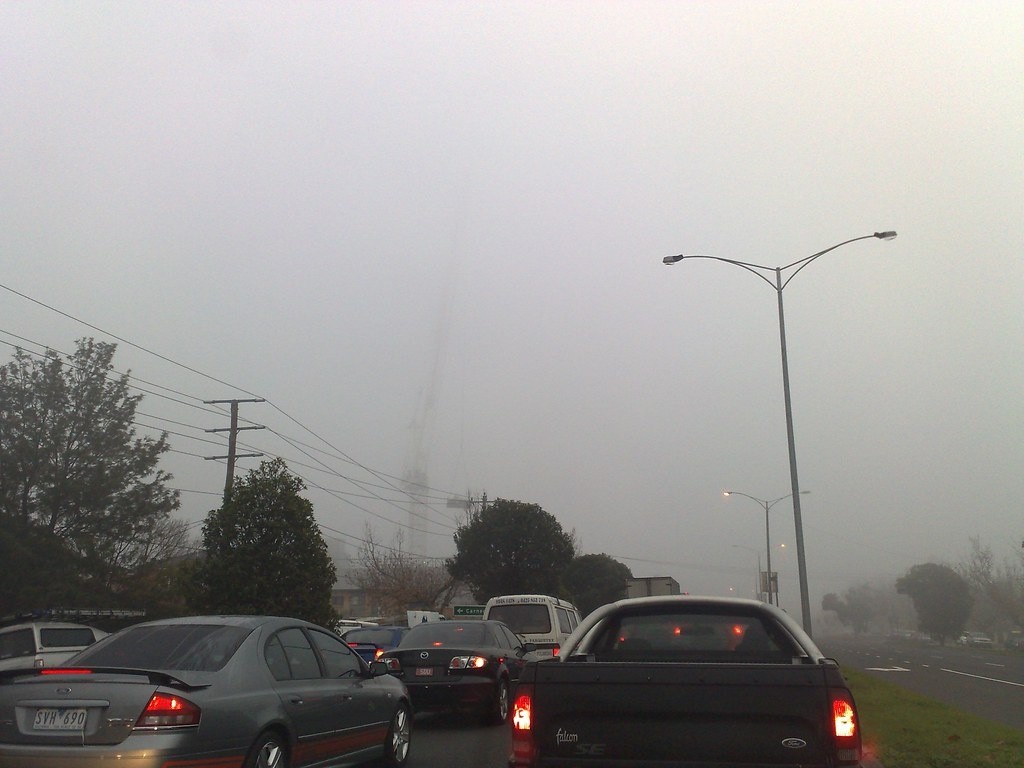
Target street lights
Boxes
[731,543,785,604]
[665,232,898,643]
[723,491,808,603]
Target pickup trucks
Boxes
[507,595,865,768]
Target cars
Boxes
[900,621,994,648]
[0,608,445,665]
[374,619,537,727]
[0,614,414,768]
[339,625,411,669]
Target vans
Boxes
[482,595,585,661]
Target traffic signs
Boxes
[453,605,487,616]
